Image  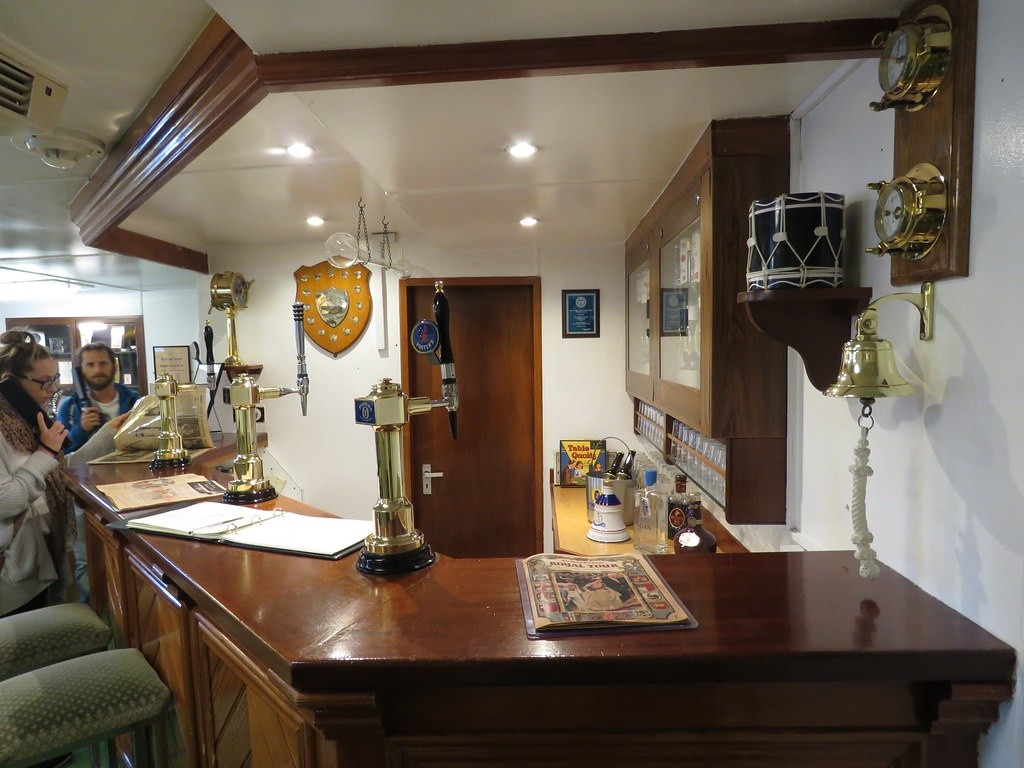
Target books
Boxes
[522,553,689,631]
[103,499,377,561]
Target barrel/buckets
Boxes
[586,437,634,526]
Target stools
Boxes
[0,604,170,768]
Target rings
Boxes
[57,430,62,433]
[90,418,94,421]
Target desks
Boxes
[550,468,751,555]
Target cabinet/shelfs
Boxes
[624,114,791,526]
[5,316,148,398]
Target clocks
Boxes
[864,163,947,260]
[870,3,951,113]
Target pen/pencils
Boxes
[189,516,245,534]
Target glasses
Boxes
[16,373,61,391]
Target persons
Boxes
[0,329,78,619]
[57,343,142,456]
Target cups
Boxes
[672,420,726,470]
[638,401,664,428]
[633,451,671,496]
[671,440,726,506]
[638,417,663,448]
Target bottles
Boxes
[634,470,668,556]
[602,451,624,480]
[674,492,717,553]
[668,475,689,540]
[614,448,636,480]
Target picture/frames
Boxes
[49,338,64,353]
